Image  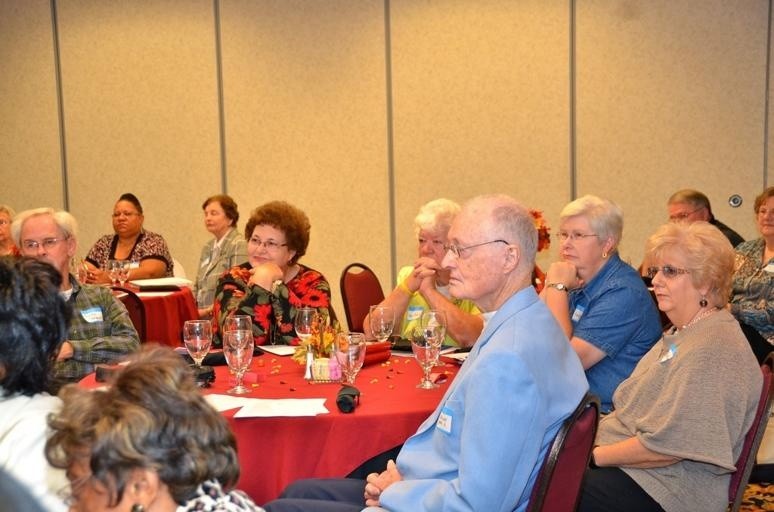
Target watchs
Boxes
[547,281,569,294]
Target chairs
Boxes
[526,395,600,510]
[340,262,385,333]
[728,351,774,512]
[107,286,146,344]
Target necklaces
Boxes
[682,307,719,328]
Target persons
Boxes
[10,207,142,384]
[79,193,173,284]
[1,255,74,512]
[209,201,331,349]
[539,195,659,416]
[262,195,594,512]
[43,345,266,511]
[575,220,764,510]
[0,204,21,257]
[364,198,483,350]
[725,187,774,366]
[638,190,745,248]
[191,194,248,319]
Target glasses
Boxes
[111,210,142,221]
[555,231,599,243]
[54,468,96,510]
[17,238,70,253]
[646,265,695,279]
[249,238,294,253]
[669,207,705,225]
[442,239,510,260]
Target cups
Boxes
[411,326,441,389]
[420,309,448,367]
[334,333,366,382]
[369,305,395,343]
[223,331,254,395]
[184,321,213,365]
[295,307,317,339]
[114,260,131,284]
[104,260,114,284]
[224,314,252,330]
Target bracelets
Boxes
[400,279,418,299]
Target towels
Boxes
[370,335,447,357]
[192,367,219,383]
[262,323,288,348]
[454,343,471,355]
[335,383,361,413]
[177,350,231,367]
